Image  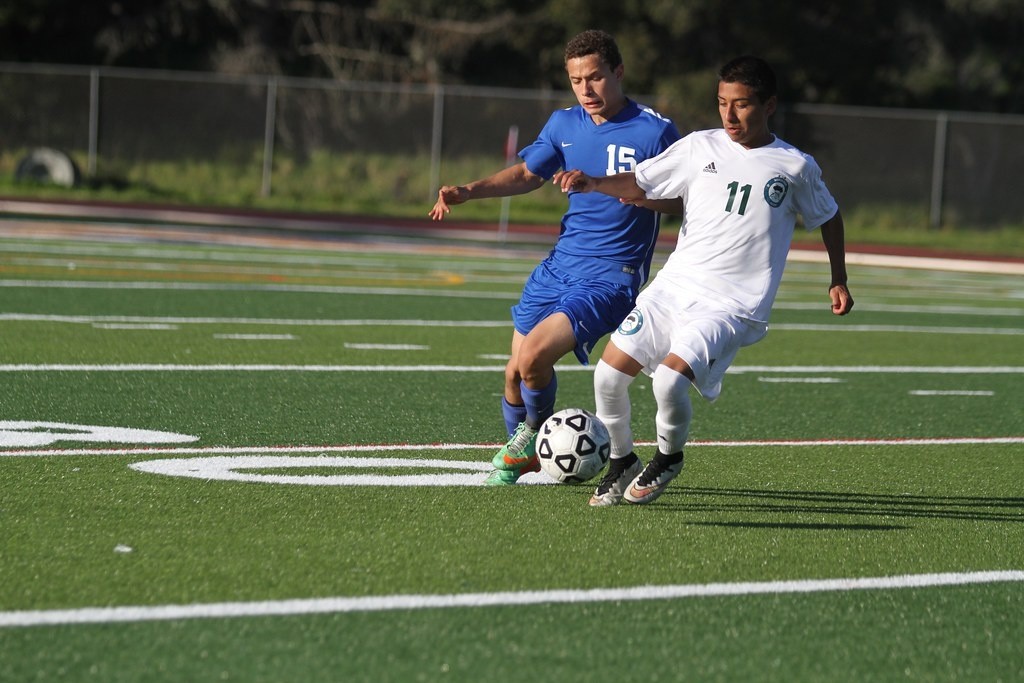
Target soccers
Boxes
[534,407,612,484]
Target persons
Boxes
[552,56,854,506]
[428,30,681,487]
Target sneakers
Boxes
[589,451,642,507]
[483,468,529,486]
[492,423,540,471]
[623,447,684,504]
